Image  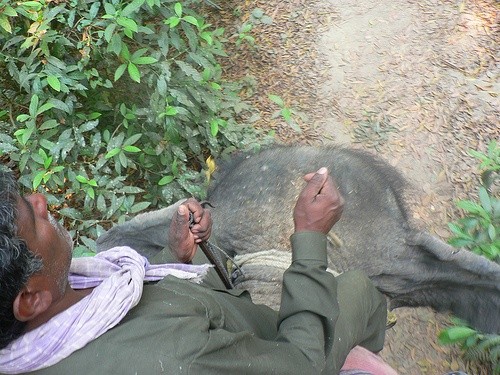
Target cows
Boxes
[94,143,500,375]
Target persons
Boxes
[0,162,390,375]
